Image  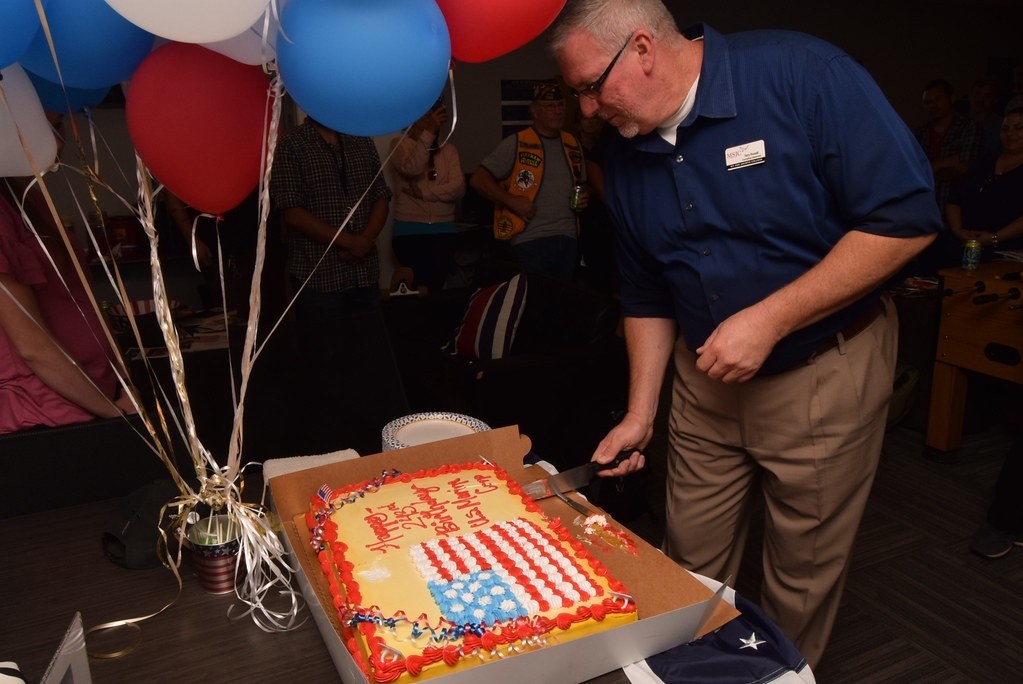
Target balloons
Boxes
[104,0,270,44]
[0,0,154,177]
[197,0,278,65]
[276,0,451,136]
[435,0,566,62]
[127,42,281,219]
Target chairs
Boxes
[0,412,148,516]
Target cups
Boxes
[185,514,245,594]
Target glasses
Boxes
[571,33,634,101]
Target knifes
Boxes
[519,449,642,500]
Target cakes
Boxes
[305,462,637,684]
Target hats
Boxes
[532,83,564,101]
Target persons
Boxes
[1,115,140,434]
[914,79,1023,387]
[153,97,467,444]
[970,429,1023,559]
[553,0,939,674]
[471,82,621,348]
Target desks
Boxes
[0,479,817,684]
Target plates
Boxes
[382,411,493,452]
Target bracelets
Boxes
[991,231,1000,246]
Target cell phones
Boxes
[432,95,446,114]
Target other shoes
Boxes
[971,528,1014,558]
[1015,535,1023,546]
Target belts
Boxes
[759,300,884,378]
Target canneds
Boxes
[99,301,113,322]
[569,184,585,213]
[962,239,981,271]
